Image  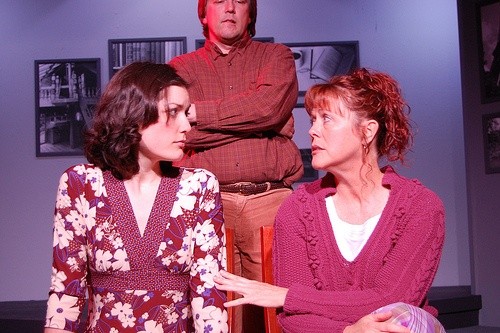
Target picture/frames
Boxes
[108,37,187,78]
[196,37,274,51]
[281,40,360,108]
[34,58,101,157]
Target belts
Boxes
[219,182,286,195]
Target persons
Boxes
[42,62,230,333]
[214,69,445,333]
[164,0,302,309]
